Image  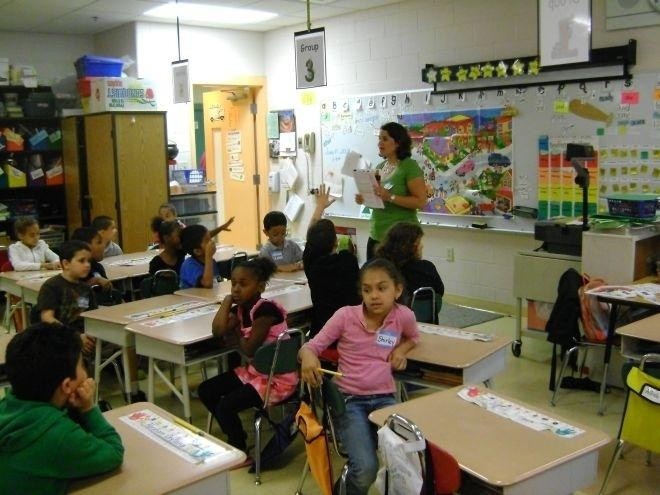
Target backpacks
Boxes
[578,273,633,344]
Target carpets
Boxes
[439,301,504,328]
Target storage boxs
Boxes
[172,170,204,184]
[91,77,157,112]
[74,55,124,79]
[178,211,218,231]
[527,301,555,332]
[0,57,9,86]
[24,93,56,116]
[9,64,38,87]
[76,77,94,97]
[172,192,218,215]
[80,97,90,113]
[607,197,658,218]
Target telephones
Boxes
[303,132,315,189]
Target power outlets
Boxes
[446,247,453,261]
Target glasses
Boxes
[268,230,288,240]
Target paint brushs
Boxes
[147,307,187,318]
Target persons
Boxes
[158,202,187,229]
[71,226,123,307]
[8,215,62,270]
[0,321,126,495]
[147,214,235,276]
[297,258,419,495]
[146,215,167,250]
[179,225,220,288]
[197,256,300,471]
[91,215,124,257]
[374,219,445,326]
[354,120,431,262]
[35,239,148,411]
[259,211,304,273]
[300,183,364,341]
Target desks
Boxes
[79,295,220,423]
[368,384,611,494]
[261,284,312,329]
[17,265,143,330]
[616,312,660,361]
[213,247,260,262]
[272,270,308,285]
[124,302,236,425]
[105,259,149,300]
[403,323,515,389]
[0,269,63,334]
[100,250,158,266]
[216,243,234,249]
[73,400,248,493]
[174,278,295,302]
[586,276,660,415]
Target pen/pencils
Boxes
[294,282,307,286]
[317,367,344,378]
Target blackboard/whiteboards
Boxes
[319,68,660,233]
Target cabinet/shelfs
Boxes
[0,86,67,251]
[511,248,581,358]
[582,224,659,317]
[60,111,170,254]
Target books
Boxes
[352,170,384,209]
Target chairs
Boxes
[551,309,652,415]
[400,287,443,325]
[206,329,305,486]
[296,376,378,495]
[600,354,660,494]
[218,251,258,280]
[384,414,460,494]
[30,303,127,413]
[141,270,208,399]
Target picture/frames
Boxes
[395,107,514,218]
[270,110,295,133]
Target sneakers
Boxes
[97,399,112,413]
[125,389,146,404]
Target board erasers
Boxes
[472,222,488,230]
